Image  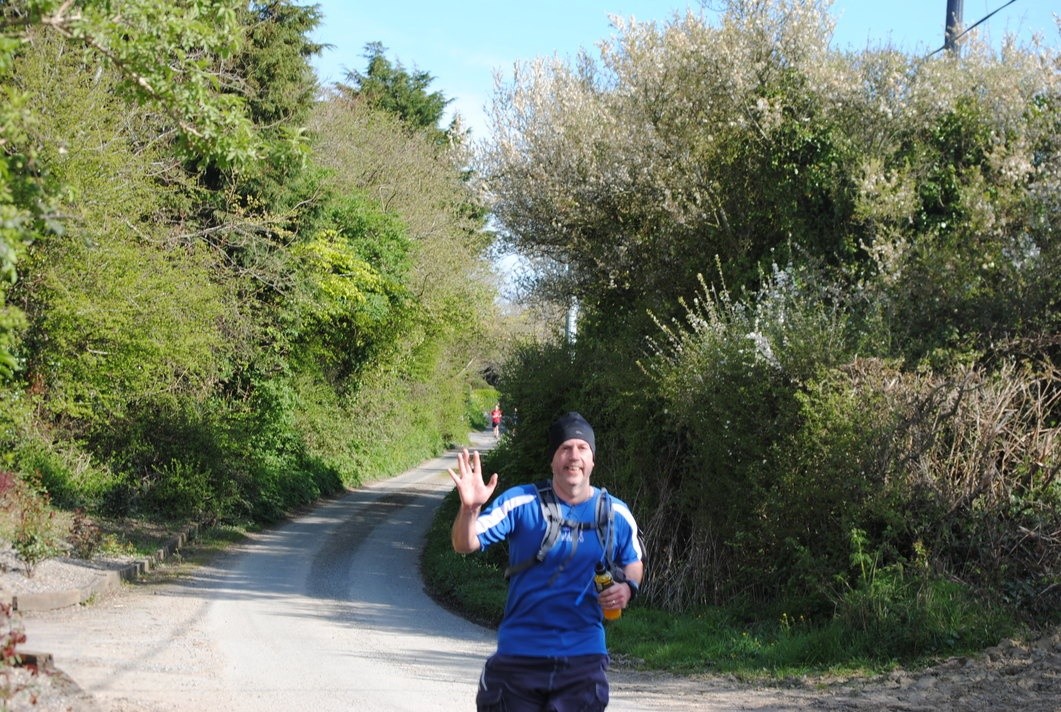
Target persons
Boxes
[491,405,502,440]
[448,412,644,712]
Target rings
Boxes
[612,600,615,605]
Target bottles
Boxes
[593,560,622,622]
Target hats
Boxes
[547,411,596,466]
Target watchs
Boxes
[621,578,639,602]
